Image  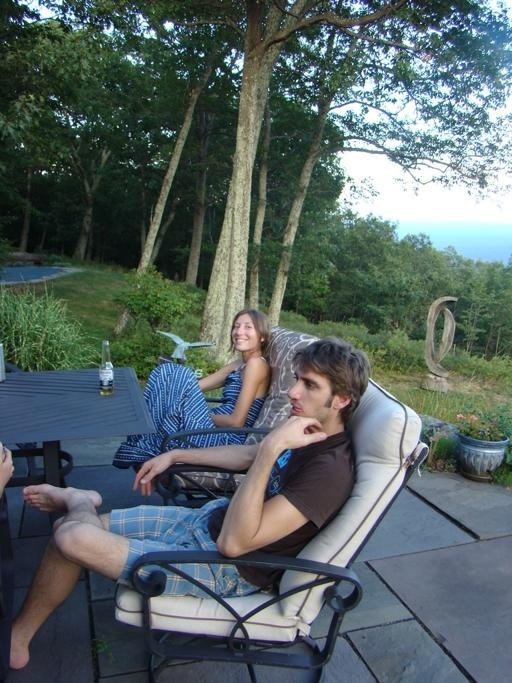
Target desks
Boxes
[1,365,157,567]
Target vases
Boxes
[457,434,509,483]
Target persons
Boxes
[113,306,276,474]
[1,443,18,495]
[8,332,371,673]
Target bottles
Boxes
[99,339,115,397]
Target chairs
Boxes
[113,378,431,681]
[132,325,322,505]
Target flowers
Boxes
[455,408,505,440]
